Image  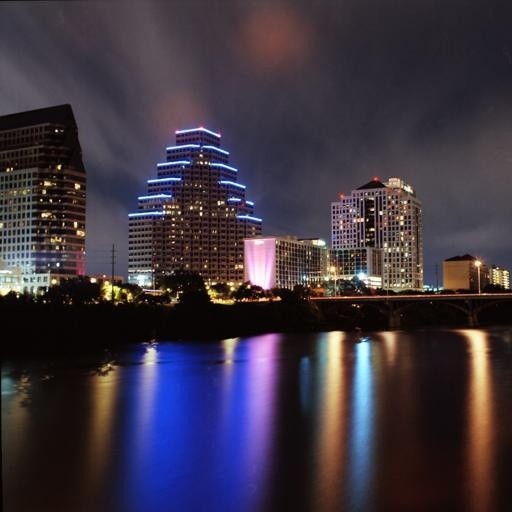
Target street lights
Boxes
[475,260,483,293]
[384,263,392,298]
[331,266,337,297]
